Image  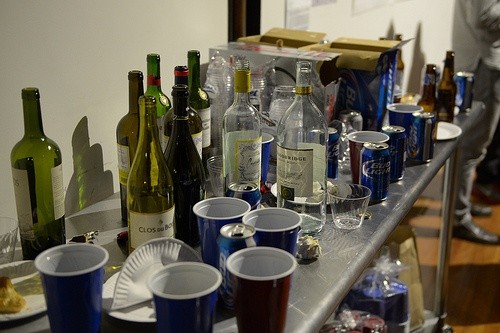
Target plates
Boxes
[436,122,463,140]
[270,179,334,205]
[100,238,203,323]
[0,260,47,322]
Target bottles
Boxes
[10,86,66,261]
[222,59,262,195]
[143,53,173,155]
[416,63,439,121]
[186,49,212,181]
[435,51,457,123]
[162,65,203,162]
[276,60,328,235]
[163,84,206,249]
[116,69,145,225]
[126,95,176,257]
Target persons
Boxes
[453,0,500,244]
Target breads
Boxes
[0,276,27,314]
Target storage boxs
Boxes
[208,28,415,132]
[378,225,426,329]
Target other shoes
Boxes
[471,204,492,217]
[452,221,500,246]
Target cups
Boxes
[347,131,389,183]
[242,207,302,288]
[226,246,297,333]
[147,261,223,333]
[0,216,19,266]
[193,197,251,269]
[386,103,424,141]
[206,61,296,200]
[327,183,371,229]
[34,243,109,333]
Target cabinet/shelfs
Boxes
[0,101,486,333]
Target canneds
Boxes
[380,125,407,181]
[407,111,436,161]
[360,142,391,202]
[224,181,261,212]
[455,72,475,113]
[217,224,259,311]
[312,128,339,180]
[339,110,363,135]
